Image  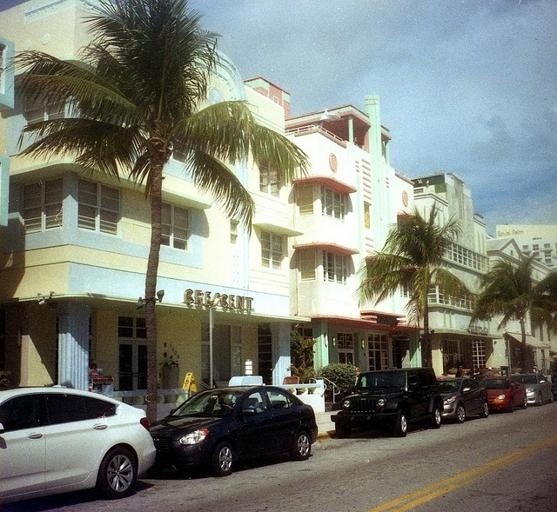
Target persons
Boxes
[87,362,102,383]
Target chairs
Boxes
[214,376,318,395]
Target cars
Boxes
[508,373,553,406]
[549,372,556,400]
[435,377,489,423]
[147,385,317,476]
[0,386,157,506]
[478,375,527,413]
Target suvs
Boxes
[330,366,443,438]
